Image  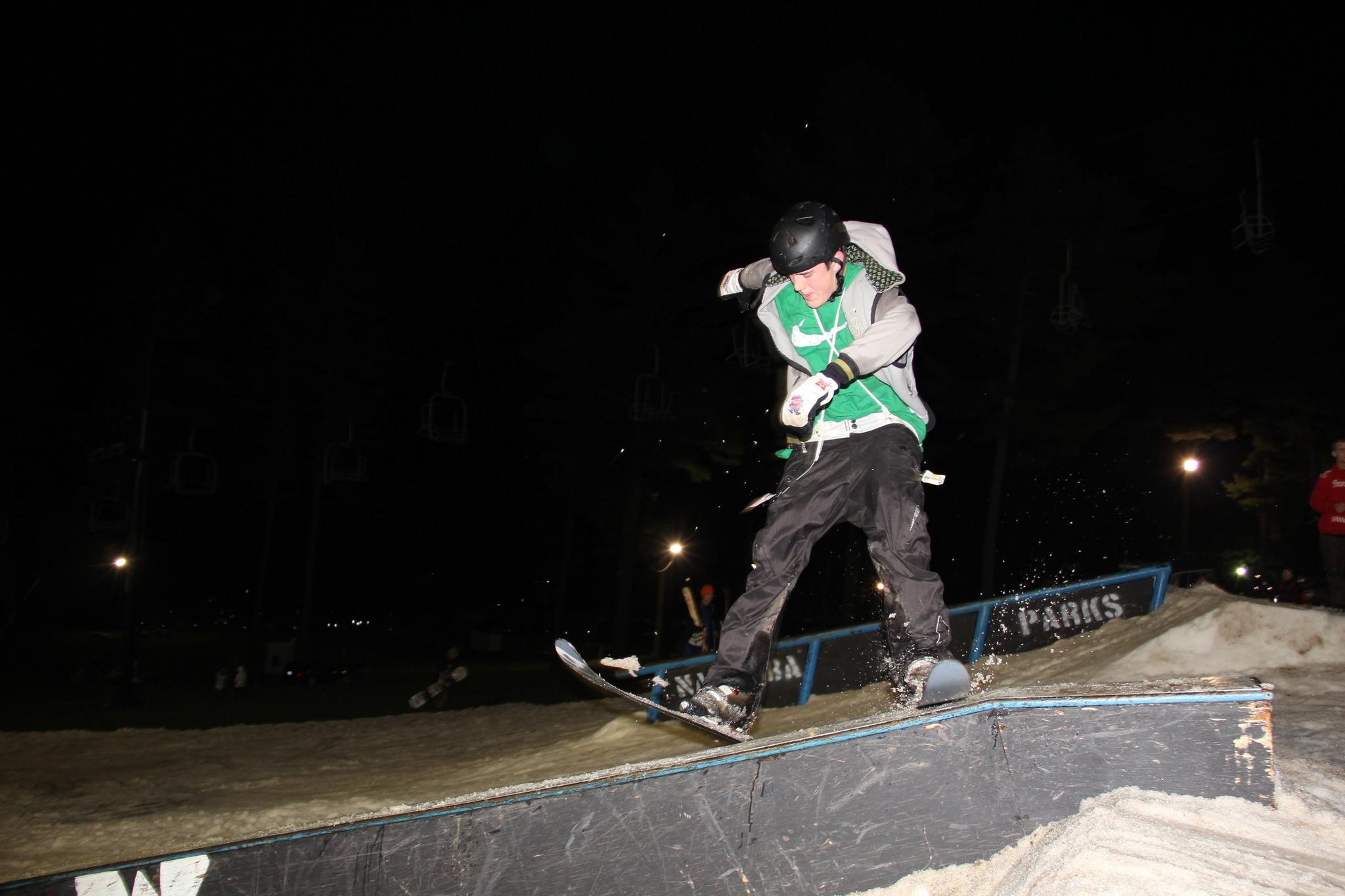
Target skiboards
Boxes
[554,637,971,744]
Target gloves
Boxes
[717,267,751,313]
[781,370,840,438]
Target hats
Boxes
[769,200,851,275]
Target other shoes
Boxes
[905,647,953,703]
[687,679,760,732]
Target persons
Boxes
[1309,439,1345,611]
[675,198,957,734]
[1276,567,1309,606]
[681,581,721,656]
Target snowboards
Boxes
[682,586,708,653]
[408,666,466,710]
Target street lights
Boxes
[667,536,709,658]
